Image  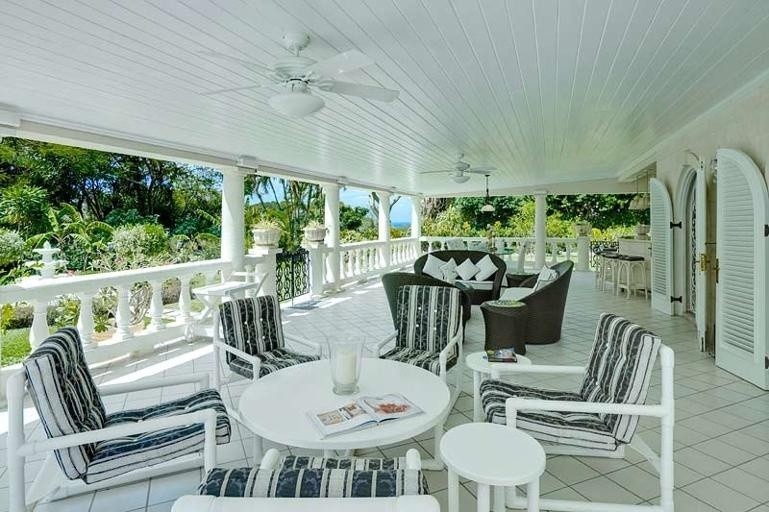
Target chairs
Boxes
[446,237,529,275]
[498,259,573,345]
[379,272,472,344]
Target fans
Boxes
[194,30,401,104]
[418,154,497,176]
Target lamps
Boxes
[628,169,650,210]
[449,171,471,184]
[265,82,325,118]
[480,175,495,212]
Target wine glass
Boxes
[326,332,366,397]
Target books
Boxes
[308,390,424,444]
[485,346,518,363]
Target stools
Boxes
[595,248,648,301]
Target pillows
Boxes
[422,254,499,283]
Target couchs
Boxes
[412,250,507,305]
